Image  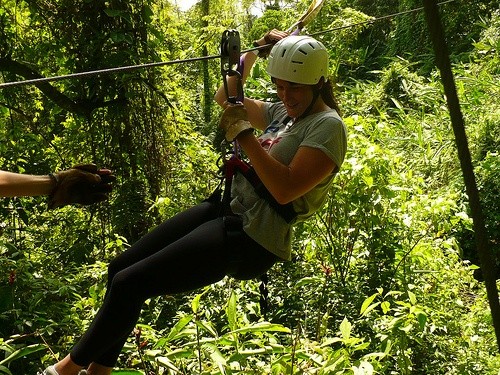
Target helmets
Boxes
[266,34,329,85]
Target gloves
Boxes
[221,100,255,142]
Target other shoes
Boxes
[37,365,59,375]
[78,369,86,375]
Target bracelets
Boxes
[249,42,262,54]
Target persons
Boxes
[42,28,347,375]
[0,164,116,211]
